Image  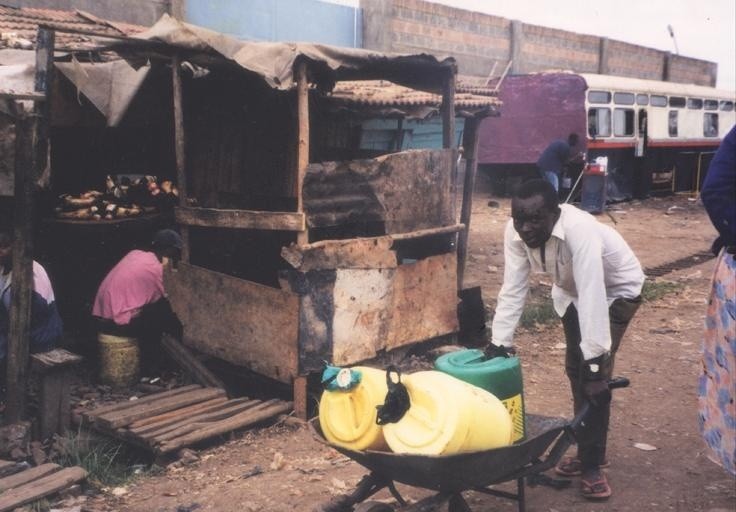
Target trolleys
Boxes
[307,376,629,512]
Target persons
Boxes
[537,133,579,199]
[0,217,185,371]
[698,125,736,482]
[484,175,645,498]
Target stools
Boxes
[31,348,81,437]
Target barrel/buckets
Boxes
[319,356,407,454]
[376,362,515,457]
[437,351,526,444]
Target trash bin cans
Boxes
[581,166,606,214]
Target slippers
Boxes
[555,456,613,477]
[578,468,614,500]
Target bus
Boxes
[499,74,736,193]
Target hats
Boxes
[157,227,189,262]
